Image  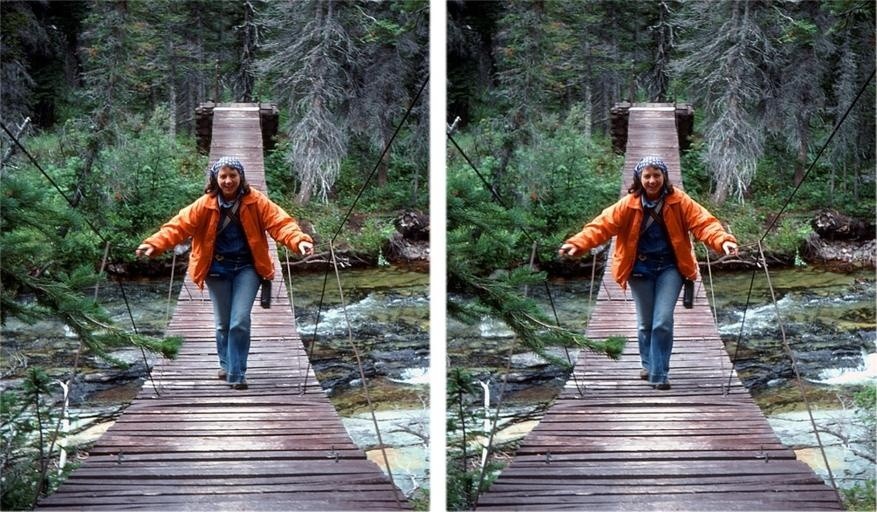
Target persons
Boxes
[559,156,742,390]
[136,156,317,389]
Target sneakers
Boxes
[218,367,227,378]
[232,381,249,389]
[640,366,651,380]
[656,381,671,390]
